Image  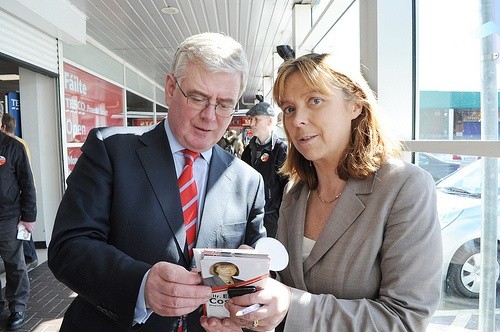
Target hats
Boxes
[246,102,275,118]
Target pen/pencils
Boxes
[236,304,260,316]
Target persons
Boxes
[226,130,244,159]
[240,102,290,238]
[203,262,243,287]
[225,52,443,332]
[0,103,37,329]
[1,114,38,271]
[46,32,267,332]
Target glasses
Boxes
[172,76,240,118]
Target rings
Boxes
[253,320,258,327]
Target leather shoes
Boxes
[25,259,39,272]
[6,311,27,329]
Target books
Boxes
[194,248,270,321]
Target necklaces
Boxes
[317,186,341,204]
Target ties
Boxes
[178,150,206,332]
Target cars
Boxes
[419,153,500,298]
